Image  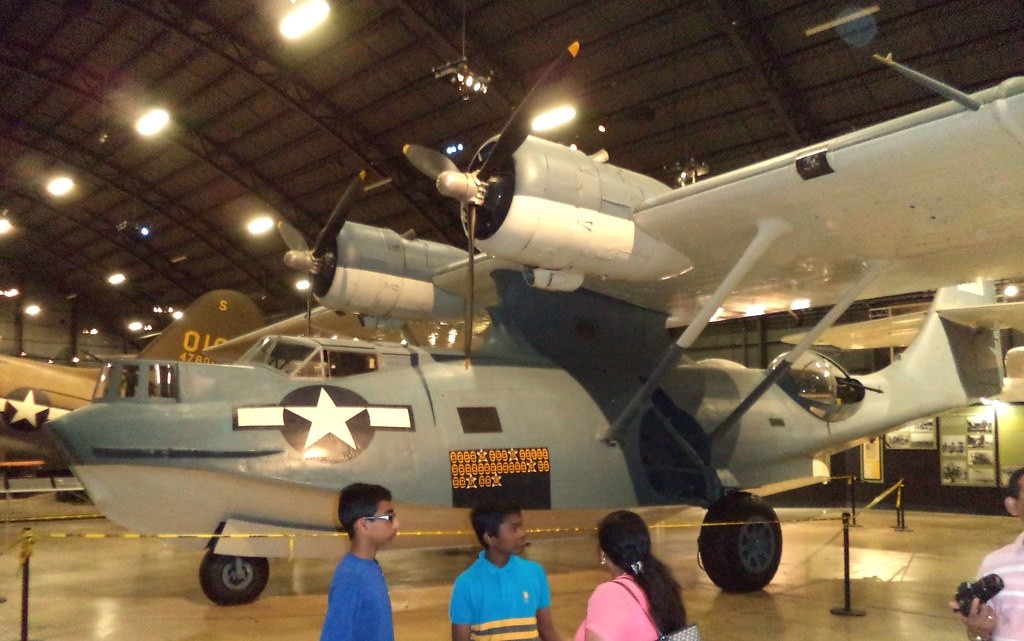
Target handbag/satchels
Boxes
[612,580,701,641]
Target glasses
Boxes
[361,513,395,525]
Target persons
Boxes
[575,511,701,641]
[948,467,1024,641]
[449,496,561,641]
[315,482,400,640]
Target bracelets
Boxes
[967,628,992,641]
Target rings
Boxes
[987,615,995,620]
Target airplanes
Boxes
[0,38,1023,606]
